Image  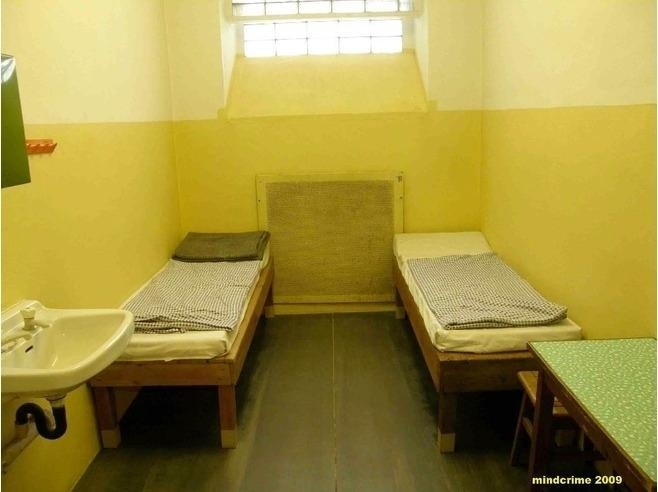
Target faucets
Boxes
[0,330,34,347]
[21,306,51,332]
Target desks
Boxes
[528,337,657,491]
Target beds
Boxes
[392,233,582,454]
[88,233,274,449]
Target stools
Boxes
[507,371,593,475]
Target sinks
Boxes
[1,297,134,396]
